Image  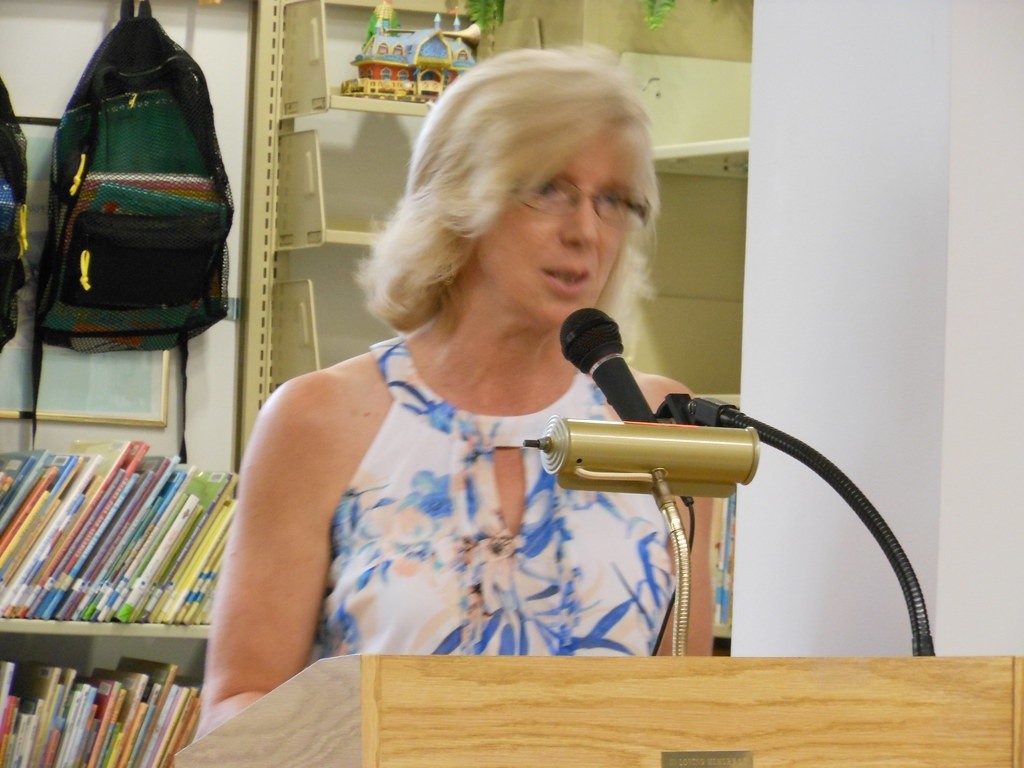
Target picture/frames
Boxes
[0,114,173,428]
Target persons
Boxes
[194,50,716,740]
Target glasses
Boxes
[516,174,652,233]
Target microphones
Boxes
[560,308,694,506]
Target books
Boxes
[0,440,240,768]
[710,485,736,626]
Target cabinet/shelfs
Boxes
[0,0,757,768]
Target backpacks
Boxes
[34,0,234,353]
[0,75,32,353]
